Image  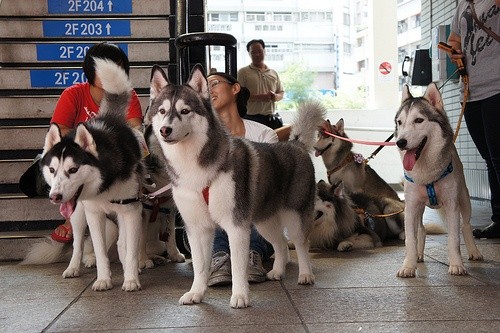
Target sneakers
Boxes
[207,250,231,286]
[248,250,267,282]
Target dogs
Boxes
[312,116,449,244]
[146,61,329,311]
[285,176,404,252]
[36,53,147,294]
[16,105,186,270]
[393,80,485,279]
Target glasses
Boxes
[208,80,233,88]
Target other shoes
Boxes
[472,221,500,238]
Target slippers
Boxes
[51,225,72,242]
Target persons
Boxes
[447,1,500,238]
[49,41,143,242]
[202,72,279,286]
[238,40,284,130]
